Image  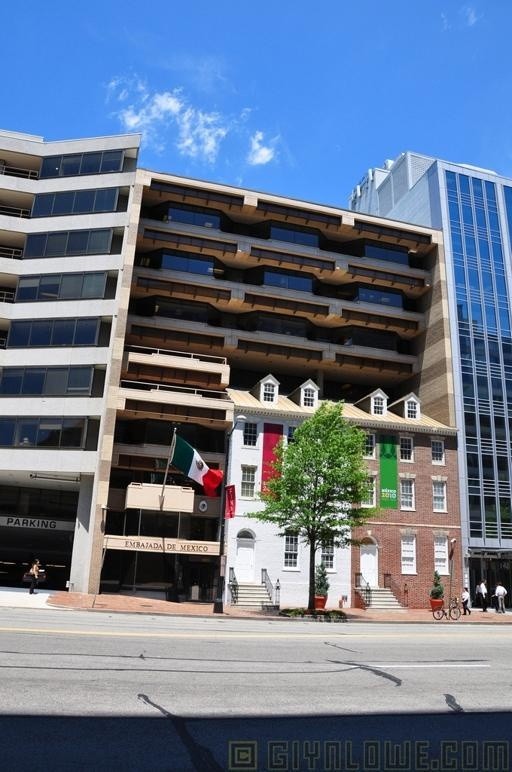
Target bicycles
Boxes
[432,595,461,621]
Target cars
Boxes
[23,569,48,585]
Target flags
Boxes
[168,436,224,498]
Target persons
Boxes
[495,582,508,613]
[461,587,471,616]
[476,581,481,607]
[493,583,499,612]
[480,579,488,612]
[29,559,40,594]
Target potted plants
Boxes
[313,561,330,609]
[428,569,445,612]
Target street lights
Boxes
[212,411,247,615]
